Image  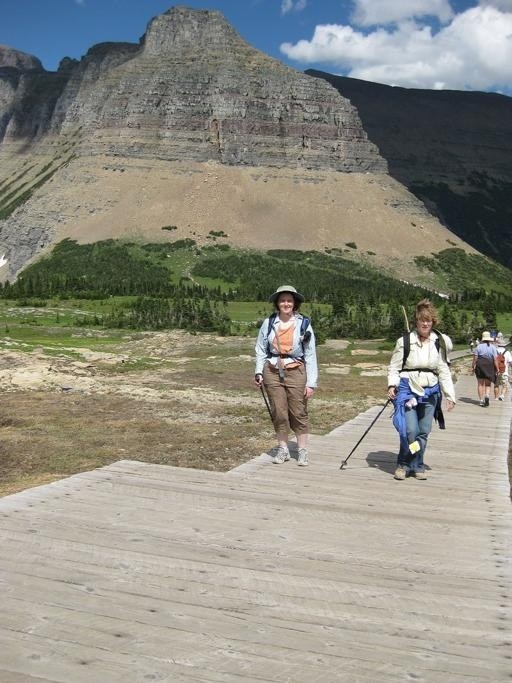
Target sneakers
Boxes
[271,447,292,465]
[479,400,484,406]
[484,395,491,407]
[414,472,428,481]
[432,328,454,365]
[393,466,408,480]
[296,447,309,466]
[498,395,505,402]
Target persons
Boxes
[495,332,511,399]
[440,329,454,369]
[471,330,500,406]
[388,297,456,480]
[254,284,318,465]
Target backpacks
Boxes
[496,348,508,374]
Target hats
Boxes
[269,284,305,305]
[497,340,506,347]
[480,331,494,342]
